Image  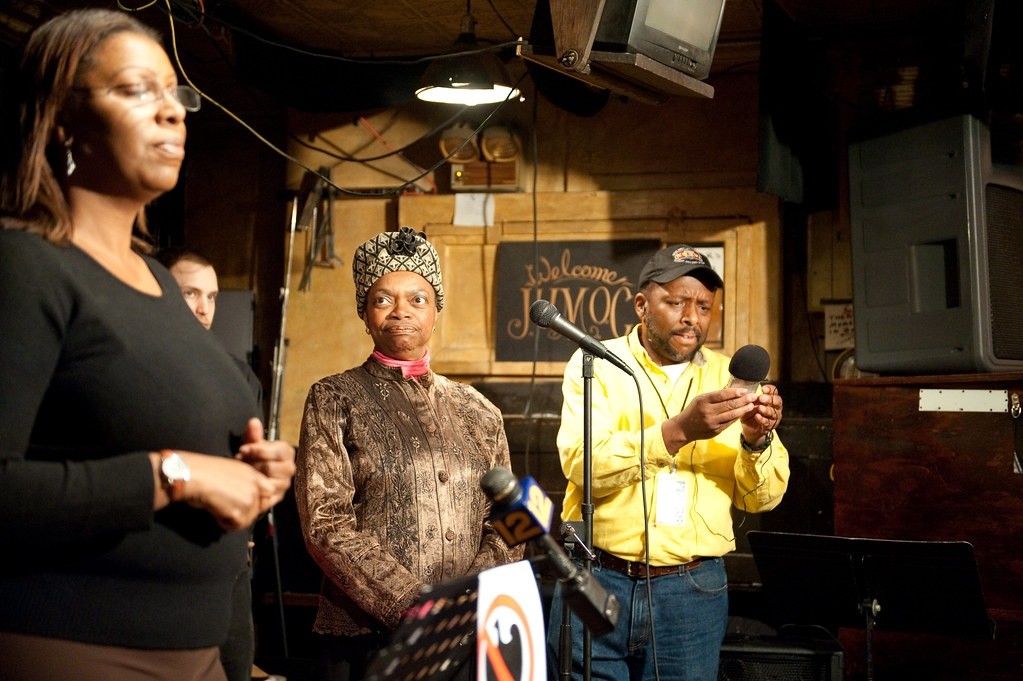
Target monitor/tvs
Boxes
[591,0,726,81]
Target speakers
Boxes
[849,114,1023,375]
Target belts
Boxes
[572,541,702,579]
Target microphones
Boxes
[723,345,772,395]
[478,465,620,638]
[529,300,634,375]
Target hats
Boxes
[637,244,724,294]
[352,227,444,320]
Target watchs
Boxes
[740,431,773,453]
[159,448,190,502]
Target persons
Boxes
[0,10,298,681]
[154,245,219,333]
[294,227,526,681]
[545,244,791,681]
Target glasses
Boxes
[70,79,201,111]
